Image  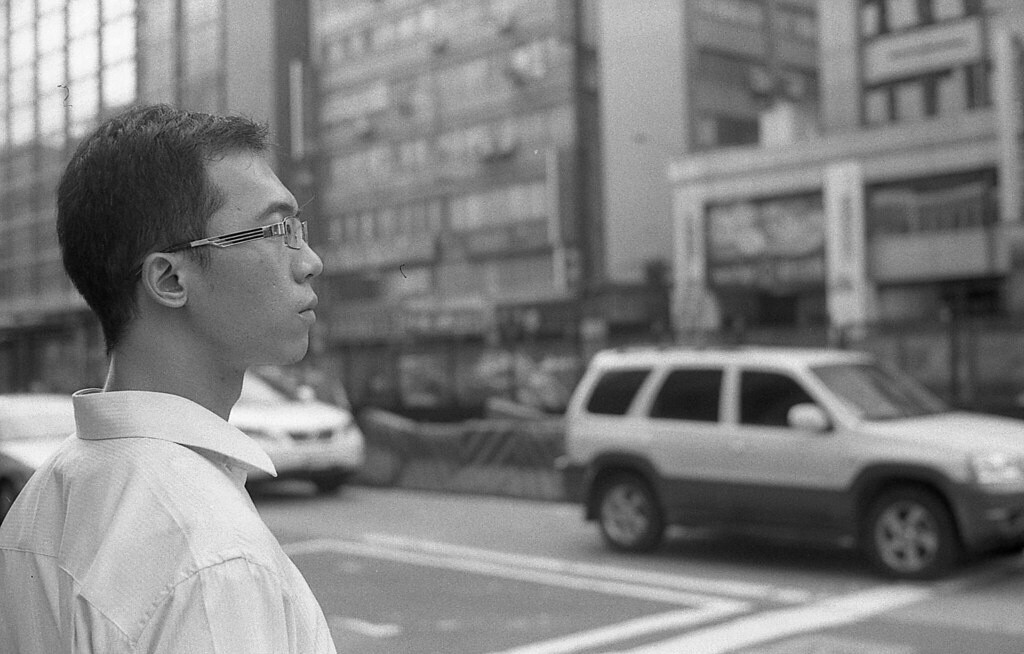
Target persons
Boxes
[0,102,337,654]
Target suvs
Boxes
[550,344,1024,581]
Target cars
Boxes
[230,373,365,499]
[0,392,76,521]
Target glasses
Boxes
[133,216,308,276]
